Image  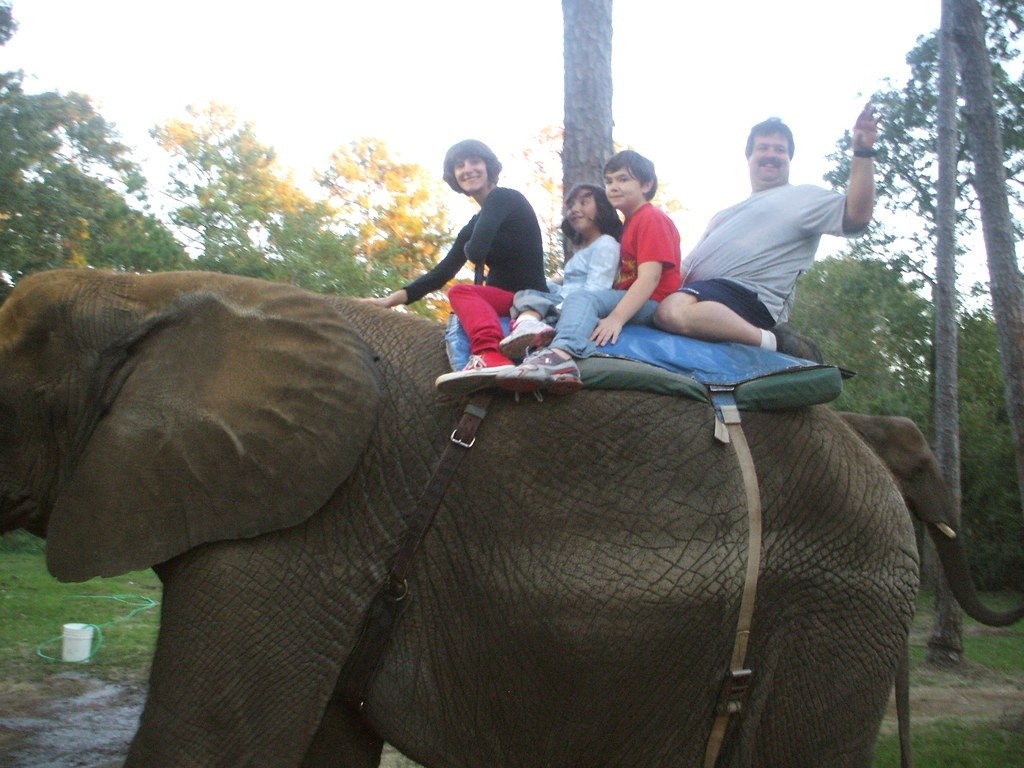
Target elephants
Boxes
[832,412,1024,628]
[0,269,921,768]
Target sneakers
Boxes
[435,348,516,398]
[498,316,556,363]
[772,322,823,365]
[495,346,584,395]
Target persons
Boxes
[358,140,551,395]
[495,150,682,395]
[652,102,884,364]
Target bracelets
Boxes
[854,150,876,157]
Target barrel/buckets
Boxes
[63,622,94,664]
